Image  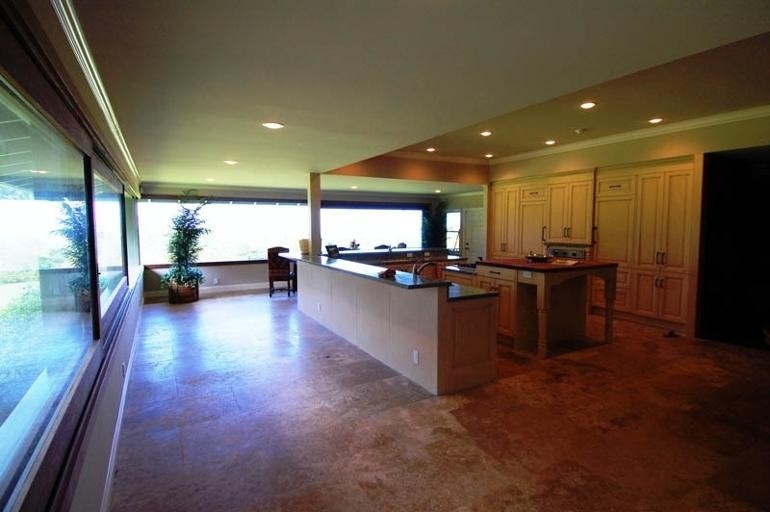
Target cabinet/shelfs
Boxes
[635,270,688,324]
[518,177,548,258]
[476,264,520,348]
[547,172,594,246]
[487,181,518,259]
[441,270,477,288]
[635,157,694,274]
[592,164,635,267]
[592,268,635,312]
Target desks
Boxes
[519,257,619,360]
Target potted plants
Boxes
[160,188,212,304]
[51,201,103,314]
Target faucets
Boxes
[388,244,396,257]
[412,262,436,274]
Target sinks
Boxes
[380,259,417,263]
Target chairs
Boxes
[267,246,296,297]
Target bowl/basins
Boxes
[525,254,553,262]
